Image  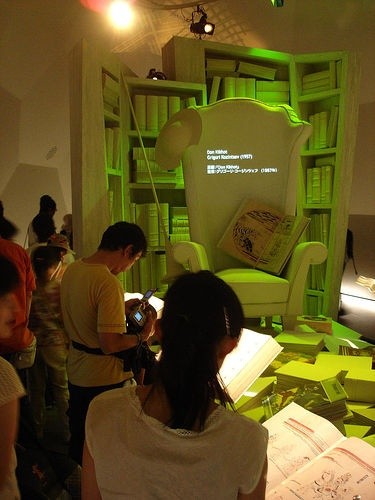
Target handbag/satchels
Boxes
[122,344,155,371]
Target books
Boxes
[119,255,168,292]
[216,199,311,275]
[306,165,334,205]
[130,203,190,248]
[133,147,184,185]
[103,73,120,111]
[133,95,196,134]
[206,59,290,105]
[105,126,121,169]
[302,60,341,95]
[309,105,339,150]
[257,402,375,500]
[307,214,330,316]
[216,328,284,404]
[124,292,164,319]
[235,312,375,436]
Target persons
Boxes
[26,214,56,257]
[81,270,269,500]
[0,201,38,365]
[0,254,28,500]
[30,234,76,442]
[28,195,57,246]
[59,221,157,467]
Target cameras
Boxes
[128,305,149,331]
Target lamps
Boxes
[189,4,215,35]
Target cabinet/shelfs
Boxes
[68,36,362,321]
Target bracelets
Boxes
[136,332,141,344]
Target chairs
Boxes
[155,97,329,330]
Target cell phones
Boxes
[131,288,157,310]
